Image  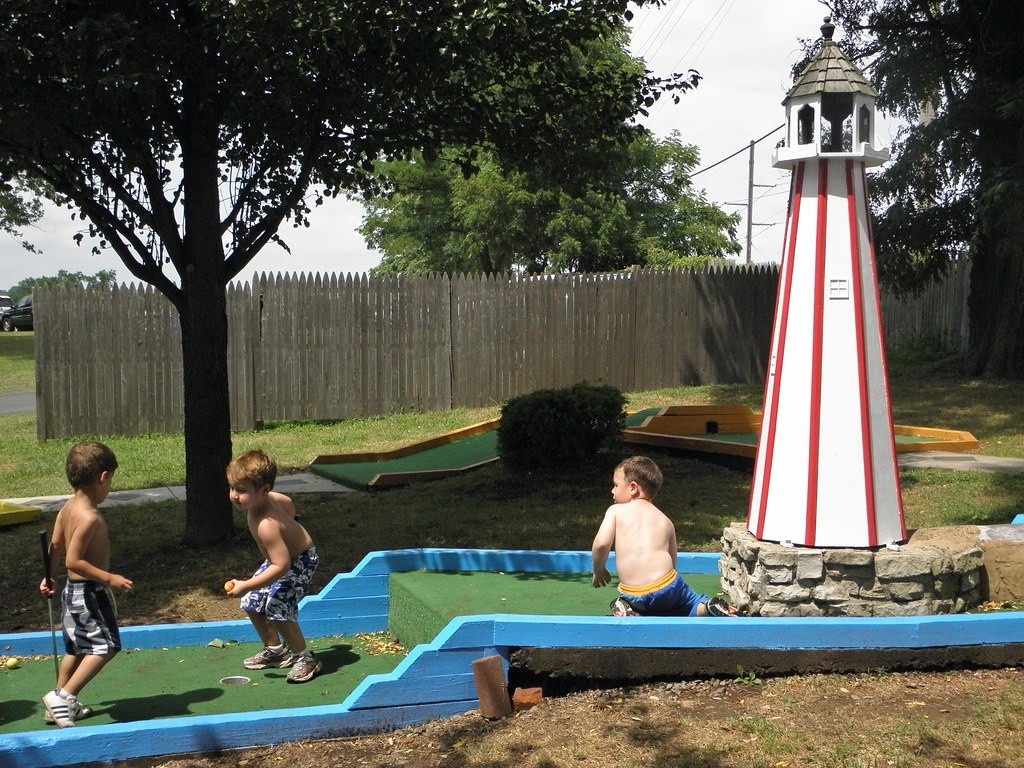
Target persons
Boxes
[593,456,742,616]
[38,439,134,729]
[223,449,324,684]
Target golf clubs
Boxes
[36,528,59,684]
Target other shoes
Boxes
[701,597,747,617]
[610,597,640,617]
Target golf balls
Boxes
[6,657,18,669]
[224,581,235,592]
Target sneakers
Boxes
[287,650,323,683]
[42,689,76,729]
[44,702,93,723]
[244,645,292,670]
